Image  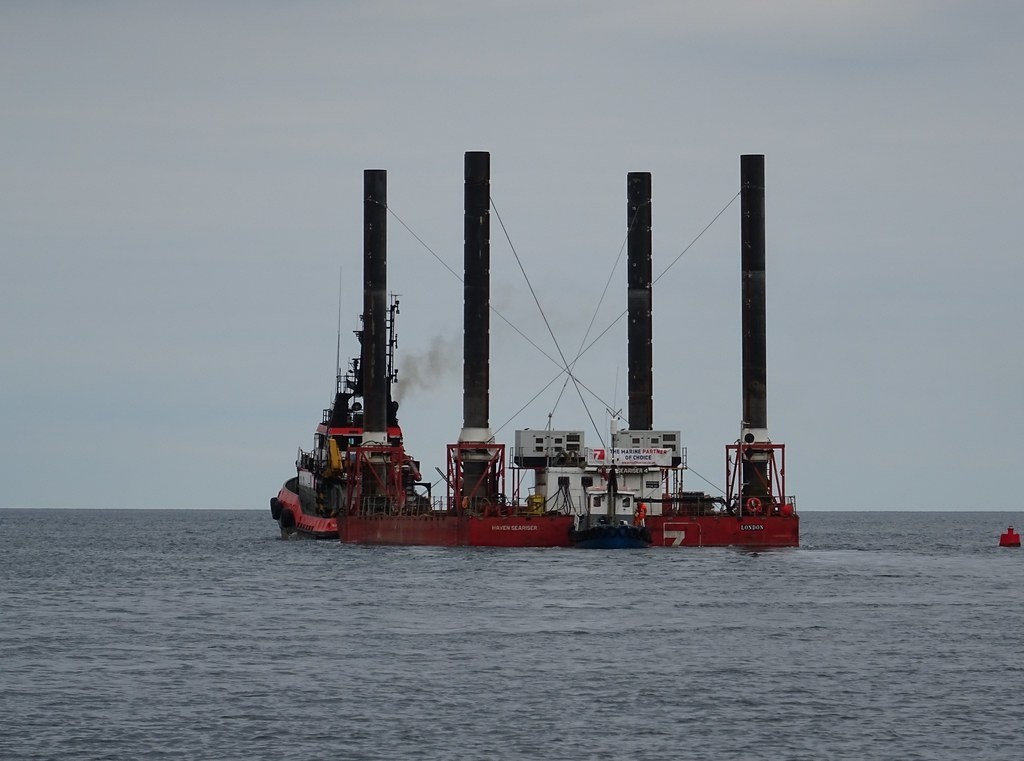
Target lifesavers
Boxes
[746,497,762,512]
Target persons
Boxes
[634,502,647,527]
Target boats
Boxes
[268,297,436,541]
